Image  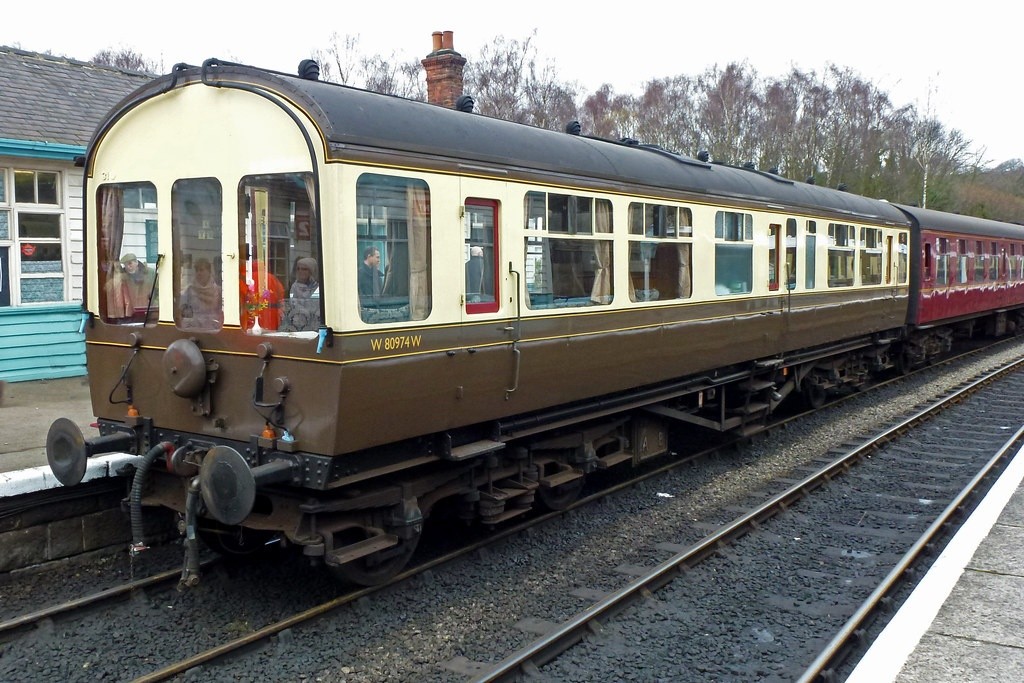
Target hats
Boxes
[120,253,136,263]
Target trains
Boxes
[44,28,1024,598]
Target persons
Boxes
[120,251,159,311]
[237,243,285,332]
[179,255,223,331]
[289,257,323,332]
[464,245,487,294]
[358,246,387,297]
